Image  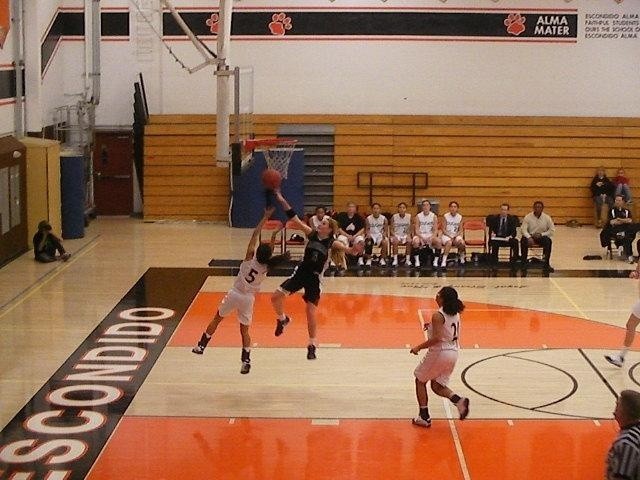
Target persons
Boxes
[388,202,414,270]
[611,166,633,205]
[32,220,71,264]
[606,193,638,264]
[308,203,334,230]
[603,390,640,479]
[332,201,366,273]
[411,198,442,273]
[269,185,364,361]
[606,246,640,368]
[520,201,556,274]
[409,286,469,427]
[440,201,467,271]
[360,202,392,271]
[191,206,286,375]
[488,203,521,270]
[590,165,614,228]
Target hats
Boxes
[38,220,50,230]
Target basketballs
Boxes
[261,169,282,188]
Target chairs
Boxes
[389,225,414,257]
[284,221,306,254]
[463,219,486,253]
[259,220,283,255]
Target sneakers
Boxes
[404,260,412,267]
[330,259,335,268]
[604,355,626,369]
[596,219,601,227]
[63,254,71,262]
[626,256,634,264]
[192,343,206,355]
[415,259,421,268]
[240,359,251,374]
[59,252,67,261]
[454,397,469,421]
[358,257,364,267]
[379,258,386,267]
[432,258,438,268]
[366,259,372,267]
[459,255,465,265]
[440,259,446,269]
[392,258,398,267]
[412,414,432,427]
[617,245,624,257]
[626,201,633,205]
[275,315,291,336]
[307,345,317,360]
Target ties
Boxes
[501,218,506,235]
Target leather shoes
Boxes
[521,268,528,277]
[543,265,554,273]
